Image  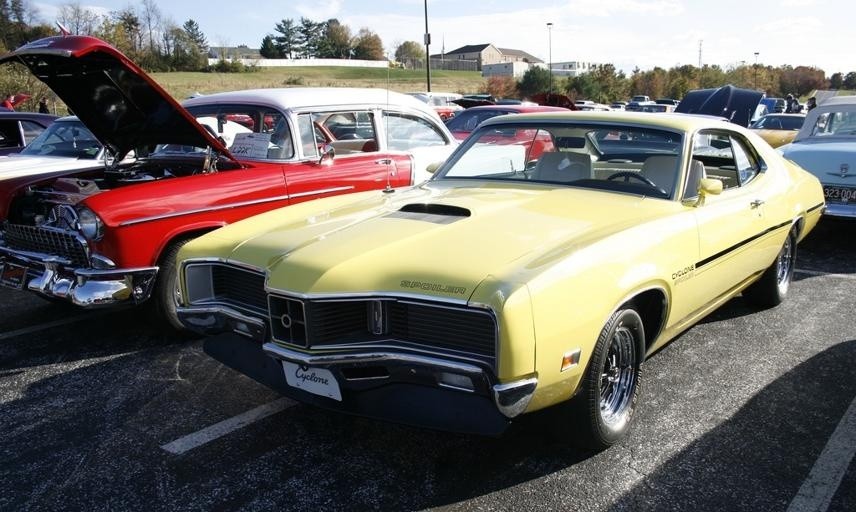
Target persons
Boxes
[37,96,50,114]
[0,94,14,110]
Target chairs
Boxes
[533,150,591,185]
[630,155,705,200]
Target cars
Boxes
[175,110,826,448]
[575,95,681,116]
[746,89,856,224]
